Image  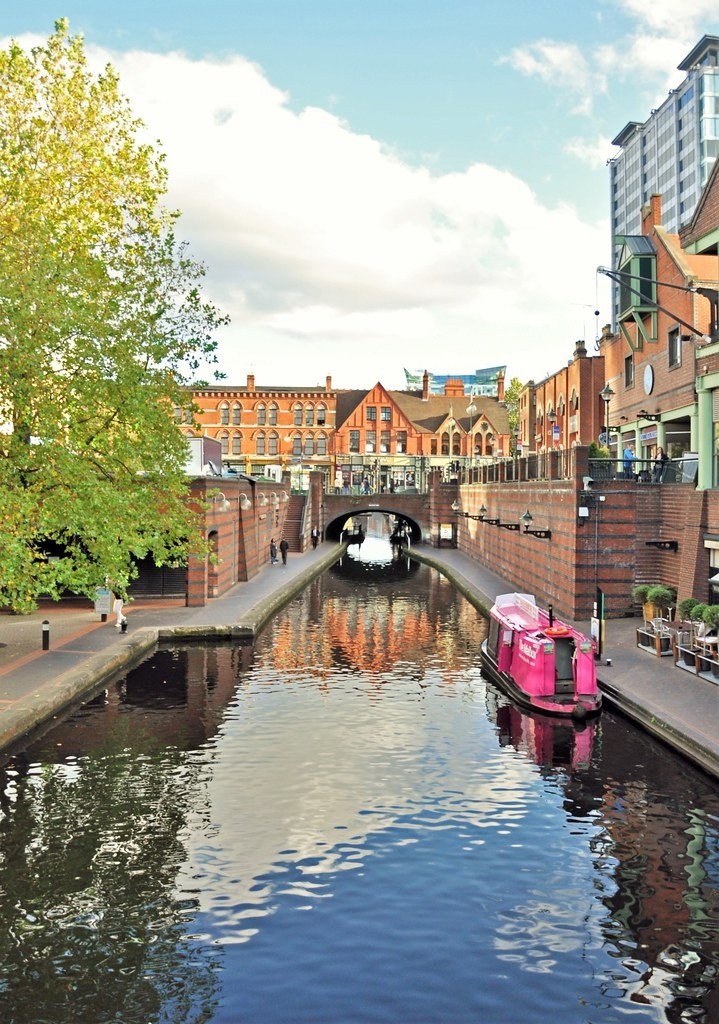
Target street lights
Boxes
[466,401,478,468]
[600,383,615,460]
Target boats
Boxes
[477,594,605,720]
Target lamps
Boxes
[269,492,279,505]
[519,510,551,540]
[280,491,290,503]
[237,493,252,511]
[214,492,230,513]
[257,492,269,507]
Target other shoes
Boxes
[273,561,277,565]
[114,623,121,627]
[122,617,127,622]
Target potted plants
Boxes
[632,584,672,653]
[678,598,719,678]
[589,441,610,479]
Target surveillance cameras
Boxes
[583,477,594,487]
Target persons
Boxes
[269,538,279,564]
[342,477,351,495]
[390,478,395,493]
[279,537,289,565]
[652,447,668,483]
[113,582,126,627]
[623,443,640,478]
[334,478,342,494]
[311,525,320,550]
[363,476,370,494]
